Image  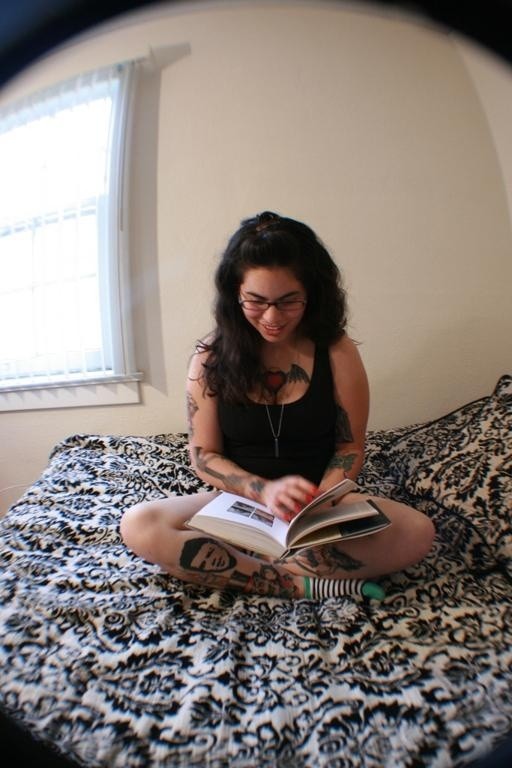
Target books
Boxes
[183,473,397,568]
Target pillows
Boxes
[376,374,512,575]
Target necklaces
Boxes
[253,339,302,462]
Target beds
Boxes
[0,423,510,768]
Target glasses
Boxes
[237,293,307,311]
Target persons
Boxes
[118,206,438,604]
[178,534,238,573]
